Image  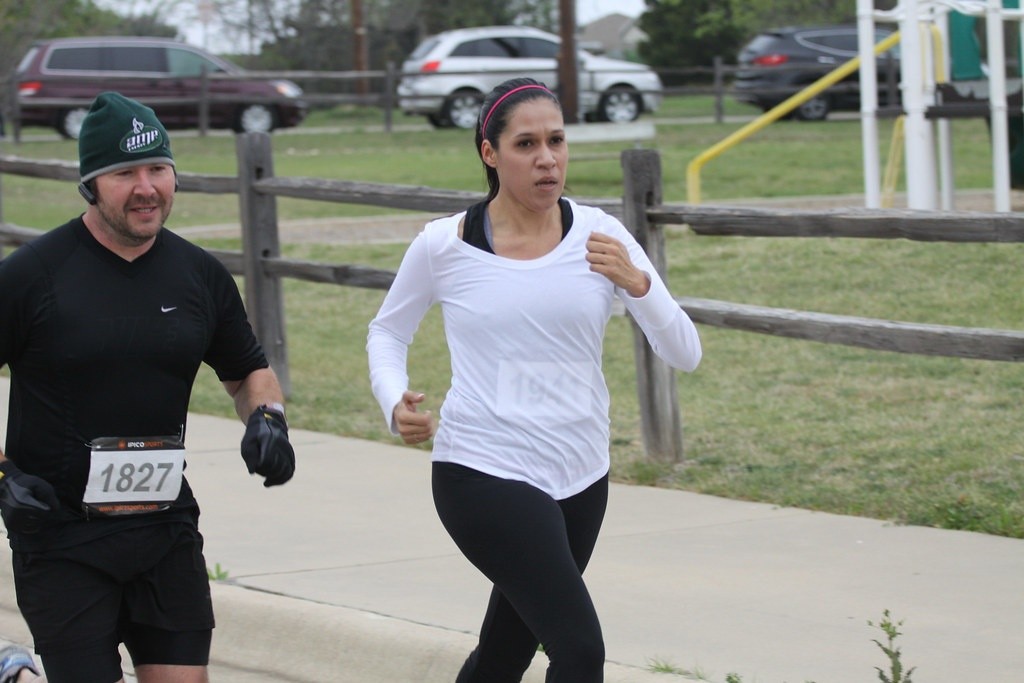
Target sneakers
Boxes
[0,641,39,683]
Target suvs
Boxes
[736,21,905,125]
[16,36,308,143]
[398,25,666,127]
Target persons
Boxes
[366,79,704,683]
[1,91,296,681]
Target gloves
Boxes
[241,407,296,486]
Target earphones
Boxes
[175,176,178,192]
[78,183,95,205]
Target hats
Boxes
[79,92,175,183]
